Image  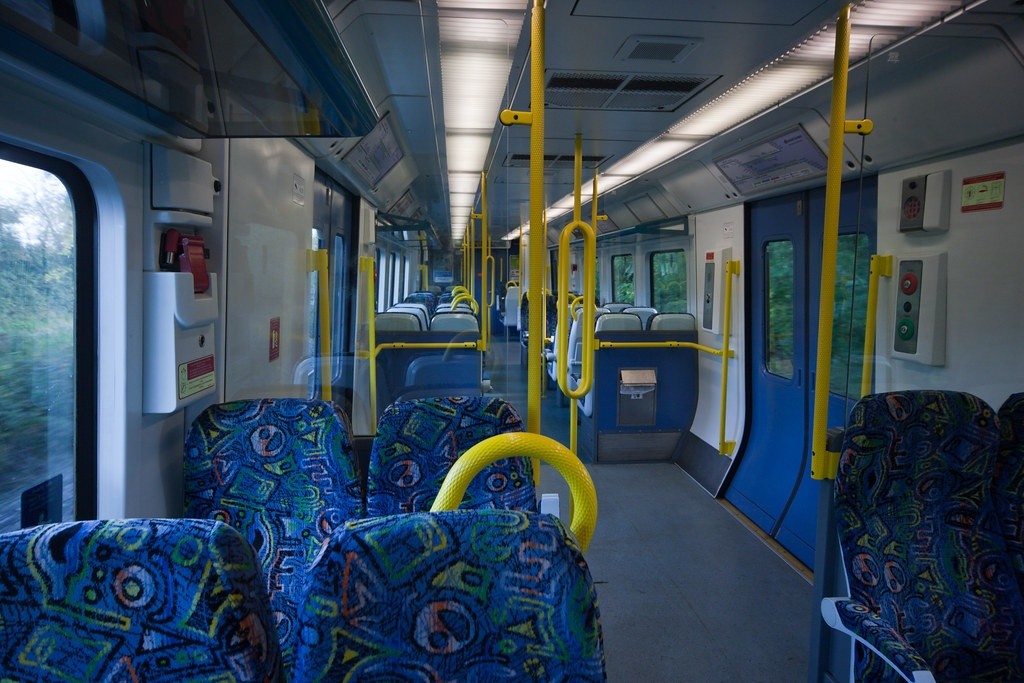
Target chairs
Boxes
[499,287,698,422]
[375,288,487,397]
[0,396,606,683]
[819,389,1024,683]
